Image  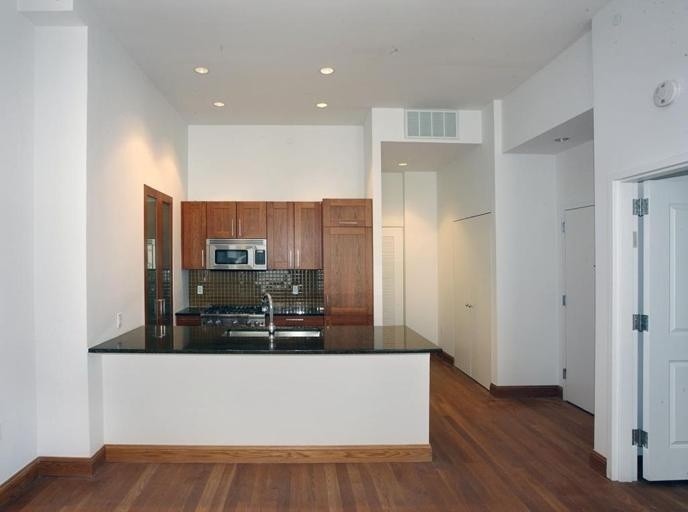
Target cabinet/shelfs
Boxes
[267,201,323,269]
[206,201,267,238]
[323,199,373,325]
[180,200,206,270]
[266,315,325,326]
[176,315,200,326]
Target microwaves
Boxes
[205,238,267,271]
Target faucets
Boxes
[261,292,276,334]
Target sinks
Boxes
[227,329,269,338]
[274,329,322,337]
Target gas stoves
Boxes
[200,304,266,314]
